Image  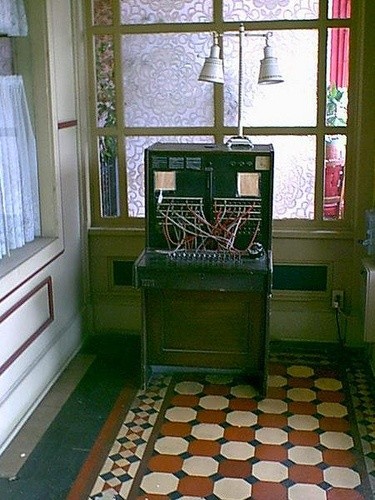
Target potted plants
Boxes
[317,83,351,217]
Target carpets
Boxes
[64,343,375,500]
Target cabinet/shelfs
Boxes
[133,251,275,399]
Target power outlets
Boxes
[330,290,344,309]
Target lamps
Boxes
[198,19,285,151]
